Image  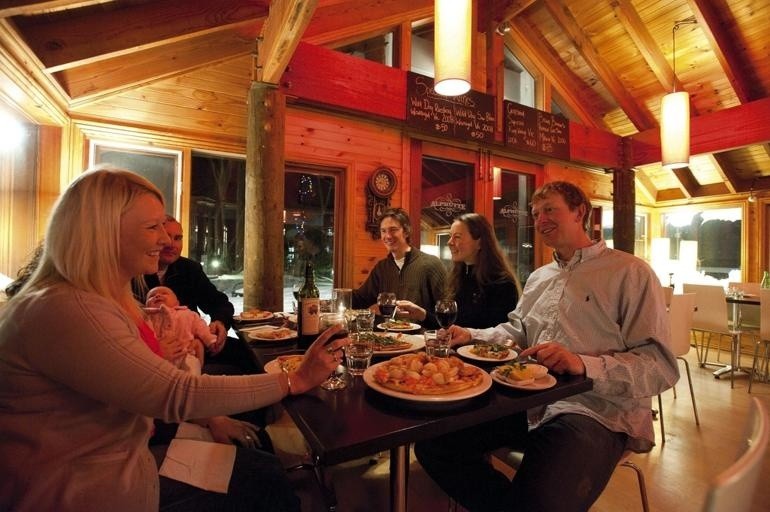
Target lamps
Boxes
[433,0,473,97]
[661,19,698,167]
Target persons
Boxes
[350,207,449,328]
[138,284,221,377]
[0,161,356,512]
[134,214,264,364]
[414,181,680,512]
[3,233,281,375]
[394,213,521,334]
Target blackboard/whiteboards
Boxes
[504,99,570,161]
[408,71,495,144]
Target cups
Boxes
[424,330,452,358]
[331,289,375,338]
[344,342,373,376]
[293,284,300,300]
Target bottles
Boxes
[297,260,320,350]
[760,271,770,288]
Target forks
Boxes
[389,305,398,323]
[503,339,538,363]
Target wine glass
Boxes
[377,292,397,322]
[724,287,744,300]
[316,314,349,391]
[435,300,458,354]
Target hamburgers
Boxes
[496,364,549,386]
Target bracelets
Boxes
[279,366,294,398]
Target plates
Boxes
[264,355,345,377]
[340,322,425,356]
[232,311,298,341]
[362,345,557,402]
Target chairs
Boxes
[621,283,770,512]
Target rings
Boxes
[326,343,333,352]
[332,352,337,361]
[246,434,252,440]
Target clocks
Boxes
[367,166,398,241]
